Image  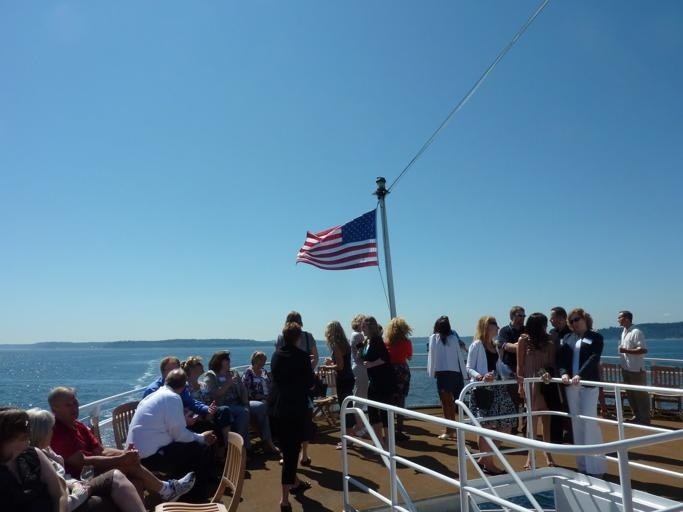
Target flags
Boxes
[295,209,378,270]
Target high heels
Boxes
[438,433,454,440]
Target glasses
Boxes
[568,317,581,324]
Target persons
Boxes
[267,310,318,510]
[465,306,606,479]
[427,314,468,440]
[1,386,196,511]
[127,349,278,498]
[324,313,414,450]
[616,310,650,426]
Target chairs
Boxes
[650,365,683,420]
[597,364,628,421]
[155,432,247,512]
[113,401,144,451]
[313,365,338,425]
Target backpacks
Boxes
[473,386,494,411]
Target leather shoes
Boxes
[627,416,650,425]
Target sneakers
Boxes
[162,472,196,501]
[337,439,355,450]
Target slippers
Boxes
[290,480,312,493]
[280,499,292,510]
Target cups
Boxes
[618,344,624,353]
[325,357,331,366]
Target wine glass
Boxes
[80,463,95,487]
